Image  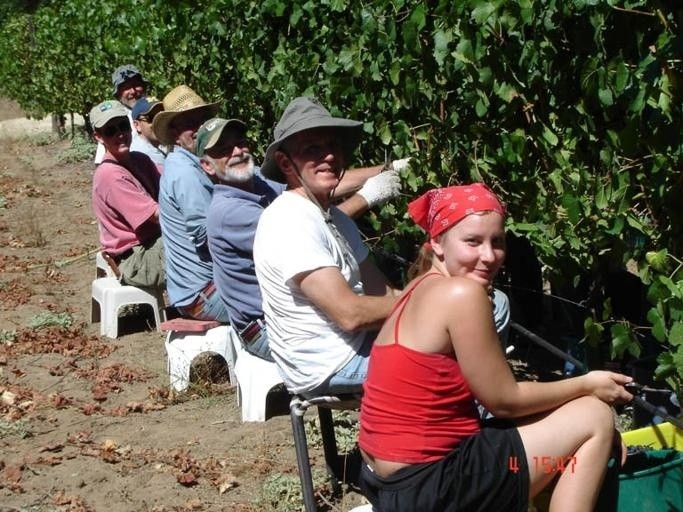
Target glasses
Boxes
[104,121,131,137]
[138,112,154,123]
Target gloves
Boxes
[358,156,420,208]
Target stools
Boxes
[289,396,364,511]
[227,329,284,422]
[95,251,116,276]
[88,278,163,338]
[164,319,233,395]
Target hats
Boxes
[131,95,163,120]
[195,116,248,157]
[260,97,365,185]
[89,100,130,132]
[111,63,149,98]
[152,83,223,145]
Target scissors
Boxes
[624,382,672,395]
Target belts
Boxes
[113,248,133,267]
[241,319,266,342]
[186,282,215,308]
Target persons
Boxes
[196,118,415,361]
[89,100,167,290]
[358,183,633,512]
[253,98,510,399]
[150,85,231,324]
[95,64,149,167]
[128,95,167,175]
